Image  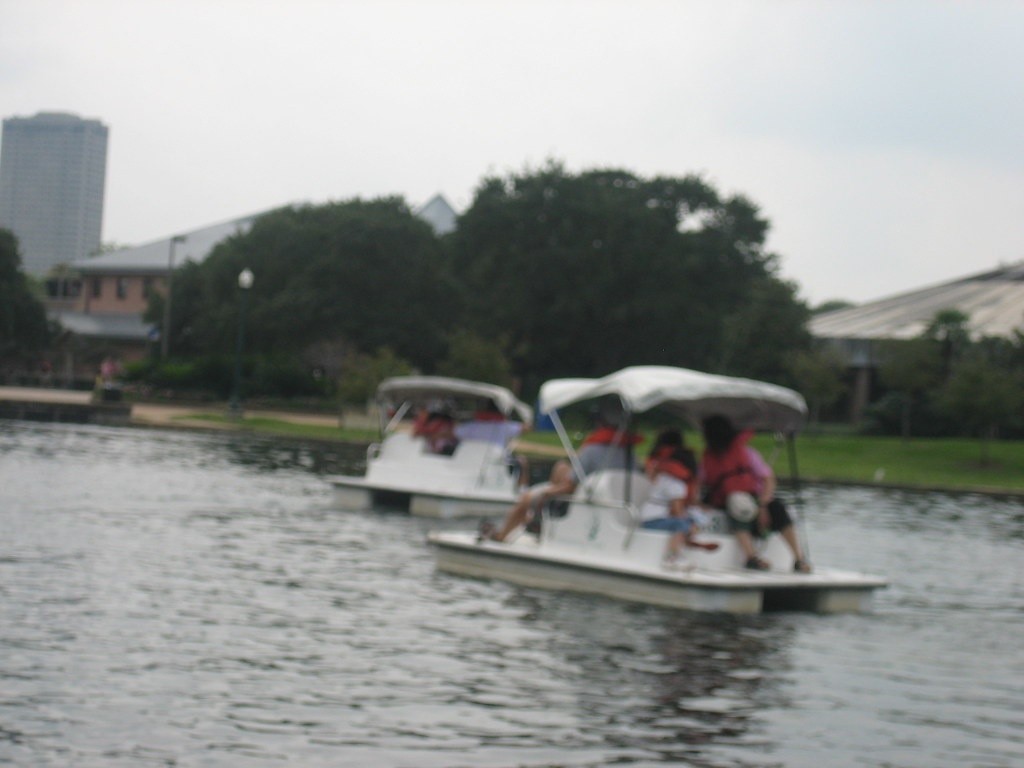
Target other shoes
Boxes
[745,556,769,571]
[794,561,810,574]
[662,552,693,568]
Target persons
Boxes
[415,396,813,576]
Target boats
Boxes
[423,363,894,619]
[321,371,577,521]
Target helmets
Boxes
[727,490,757,521]
